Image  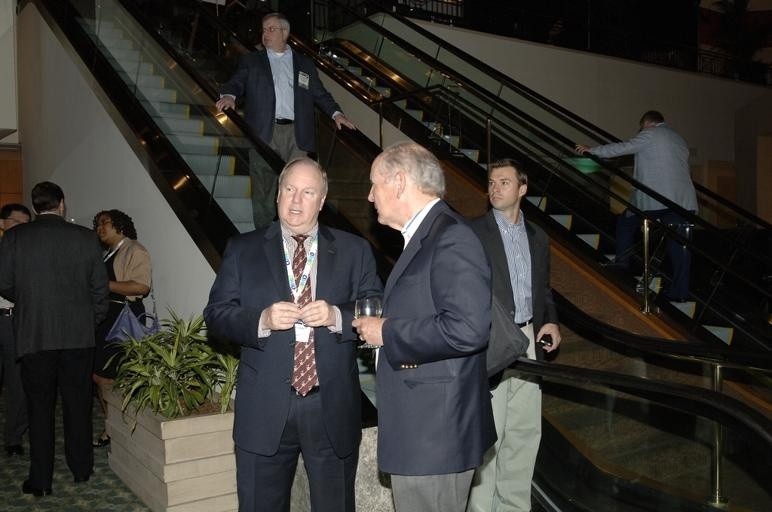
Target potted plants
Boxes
[99,302,239,511]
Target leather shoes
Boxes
[22,478,53,498]
[5,443,24,458]
[74,467,92,482]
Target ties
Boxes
[289,233,319,397]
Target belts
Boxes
[275,118,294,124]
[0,306,16,317]
[516,317,537,328]
[290,385,322,398]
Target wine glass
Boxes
[355,296,382,349]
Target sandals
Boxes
[94,430,112,447]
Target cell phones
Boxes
[540,333,552,346]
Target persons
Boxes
[467,155,563,511]
[574,109,699,304]
[202,153,384,512]
[351,139,498,511]
[0,202,32,455]
[0,180,110,498]
[91,208,151,448]
[214,12,357,230]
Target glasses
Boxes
[262,27,288,34]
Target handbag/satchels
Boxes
[104,304,162,351]
